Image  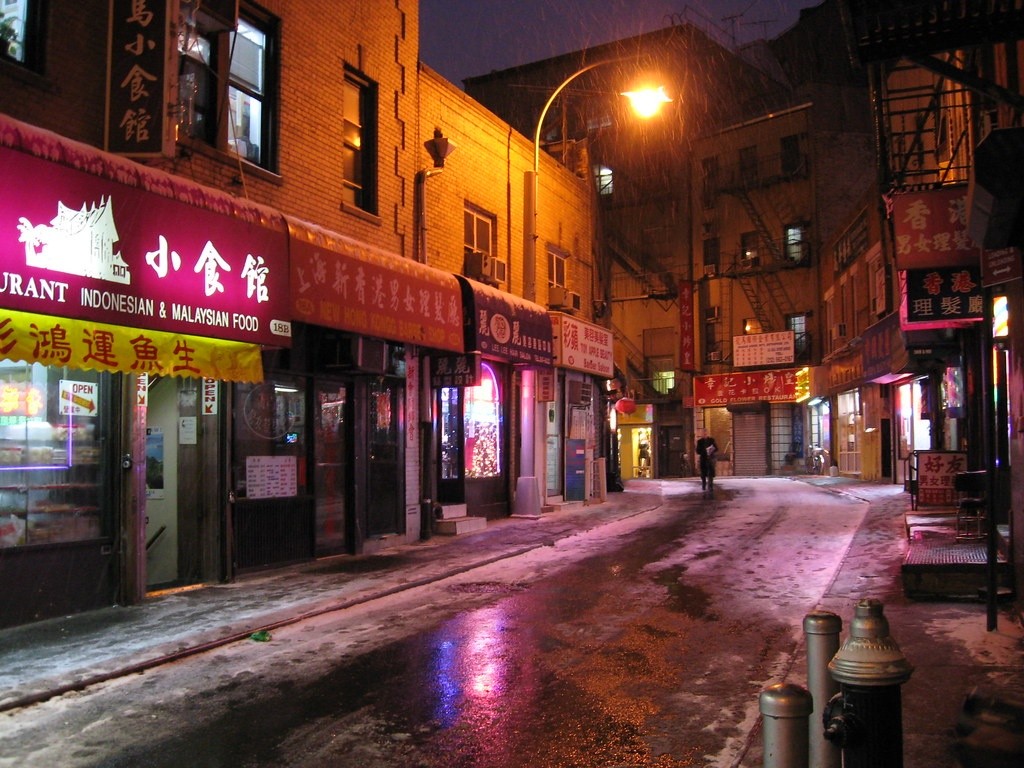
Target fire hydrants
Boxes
[822,597,914,767]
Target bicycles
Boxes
[806,444,825,475]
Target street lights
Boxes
[532,50,679,505]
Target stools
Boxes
[952,469,990,541]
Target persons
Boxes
[696,428,719,500]
